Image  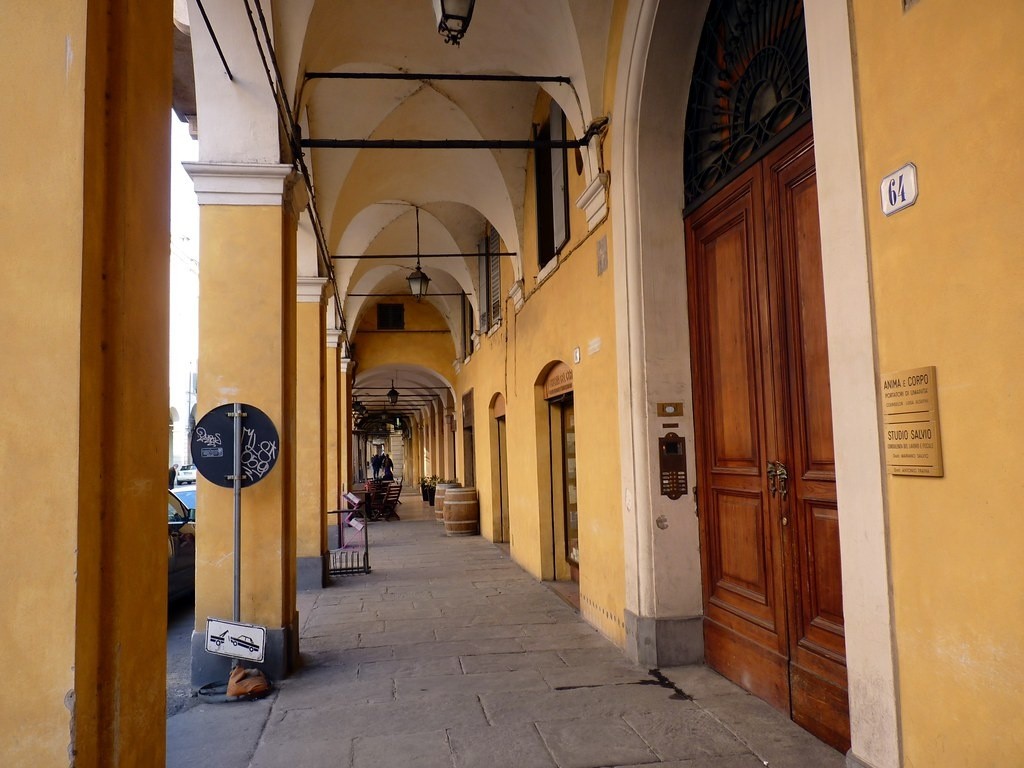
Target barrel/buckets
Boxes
[435,484,462,524]
[443,488,479,536]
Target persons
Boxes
[168,464,178,490]
[371,453,393,481]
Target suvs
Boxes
[176,464,196,485]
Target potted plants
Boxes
[419,476,439,506]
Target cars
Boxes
[170,485,196,523]
[167,490,196,627]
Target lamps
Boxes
[405,207,432,299]
[434,0,476,48]
[386,379,398,405]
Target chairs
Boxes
[352,477,402,520]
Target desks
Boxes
[354,491,382,521]
